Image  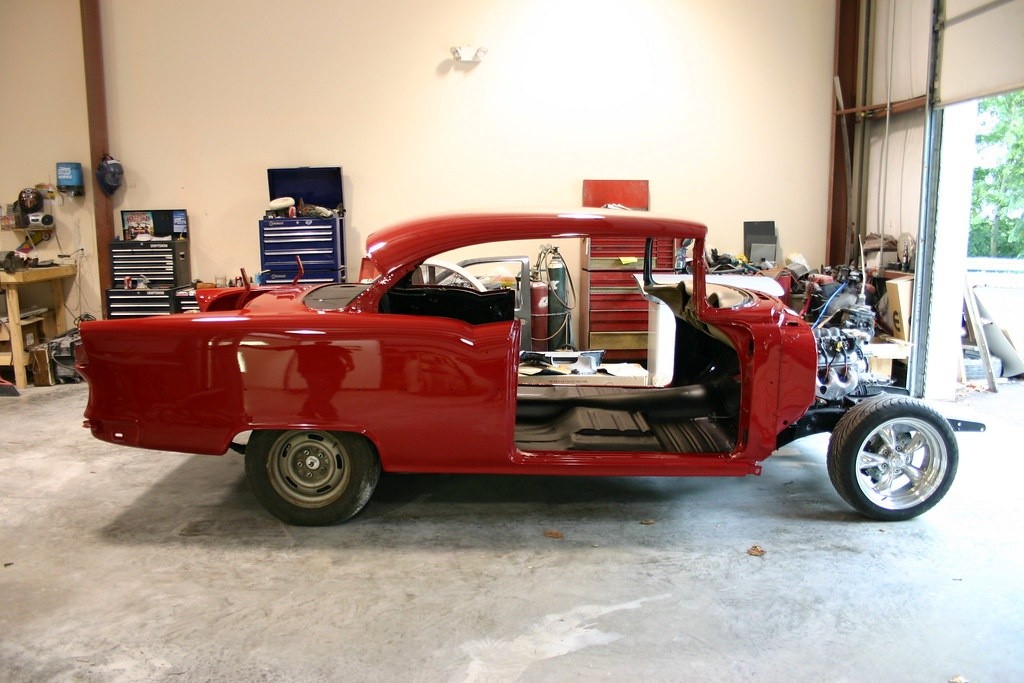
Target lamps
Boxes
[449,43,488,63]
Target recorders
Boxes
[20,212,55,228]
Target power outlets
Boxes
[79,241,87,257]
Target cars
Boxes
[83,210,987,528]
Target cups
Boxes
[215,275,225,289]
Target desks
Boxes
[0,264,78,390]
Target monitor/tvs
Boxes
[268,167,343,212]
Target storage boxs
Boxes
[885,276,914,343]
[109,209,191,290]
[0,317,45,353]
[259,165,347,272]
[260,268,347,286]
[105,283,193,319]
[589,233,684,272]
[585,271,676,334]
